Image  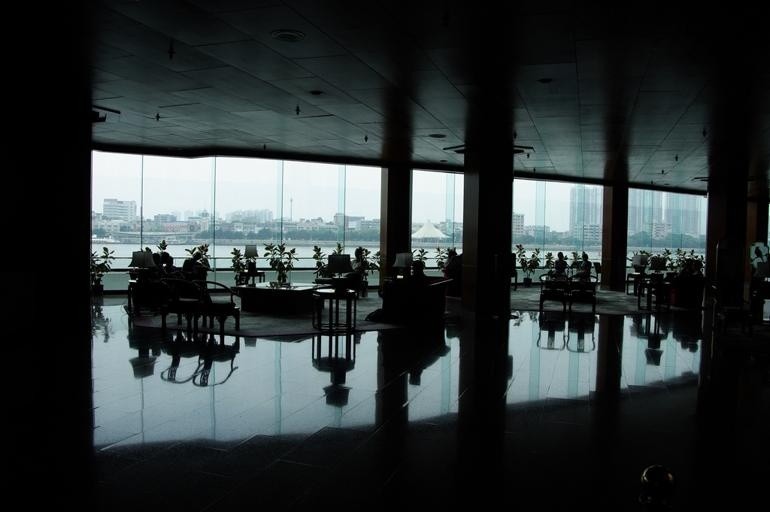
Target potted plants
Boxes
[413,251,428,274]
[519,243,539,289]
[538,268,600,312]
[89,248,110,309]
[354,247,378,296]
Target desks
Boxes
[637,314,668,338]
[627,273,666,311]
[631,254,667,280]
[308,337,358,373]
[226,280,325,318]
[312,287,360,331]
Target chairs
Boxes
[669,272,704,314]
[538,316,600,355]
[123,277,243,338]
[158,339,248,387]
[398,274,451,321]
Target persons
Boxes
[684,259,694,269]
[567,253,592,279]
[343,248,370,280]
[411,260,428,277]
[670,259,705,310]
[553,252,568,274]
[150,251,210,291]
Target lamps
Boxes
[392,252,412,276]
[243,244,261,275]
[321,253,353,291]
[645,340,663,366]
